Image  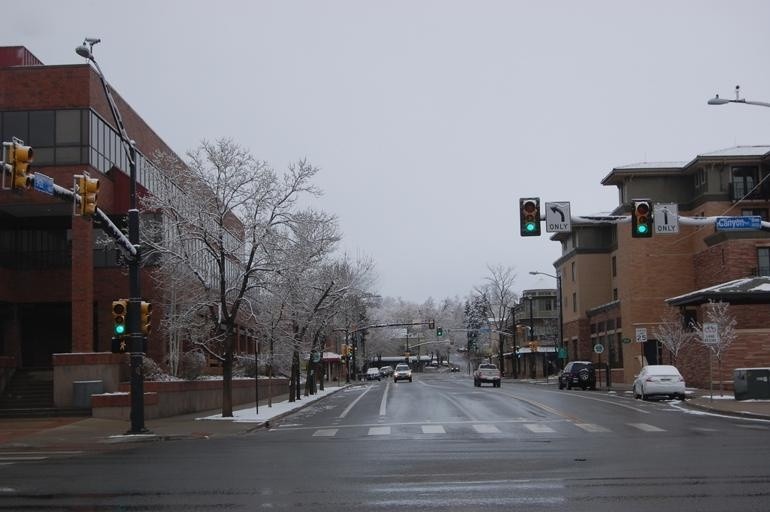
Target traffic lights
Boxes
[633,199,654,238]
[142,301,154,335]
[518,196,541,237]
[112,300,128,337]
[12,137,35,190]
[111,338,128,353]
[79,173,100,218]
[437,327,442,336]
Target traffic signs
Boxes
[716,217,763,232]
[635,328,647,342]
[703,323,718,344]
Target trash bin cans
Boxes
[734,368,770,400]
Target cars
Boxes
[432,361,438,366]
[380,366,393,377]
[367,368,380,380]
[633,365,687,401]
[442,361,448,366]
[450,367,460,372]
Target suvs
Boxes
[394,363,413,382]
[558,361,596,391]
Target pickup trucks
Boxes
[473,363,501,388]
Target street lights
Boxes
[529,271,563,371]
[76,39,152,434]
[707,95,769,109]
